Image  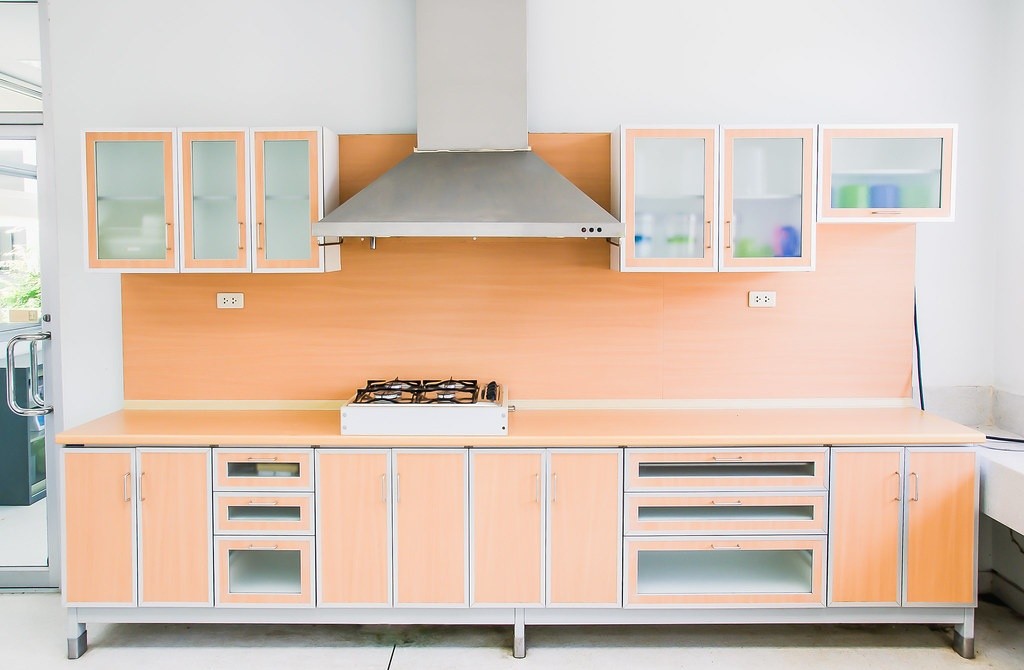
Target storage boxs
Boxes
[9,310,36,322]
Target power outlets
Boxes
[748,290,777,308]
[216,293,244,309]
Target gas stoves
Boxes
[340,376,514,436]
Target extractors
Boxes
[312,0,625,237]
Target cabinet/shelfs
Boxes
[59,448,215,607]
[819,123,957,221]
[212,447,316,610]
[178,127,342,275]
[81,128,180,273]
[828,443,981,609]
[623,447,830,610]
[0,350,47,506]
[314,448,469,609]
[610,124,817,272]
[467,448,624,609]
